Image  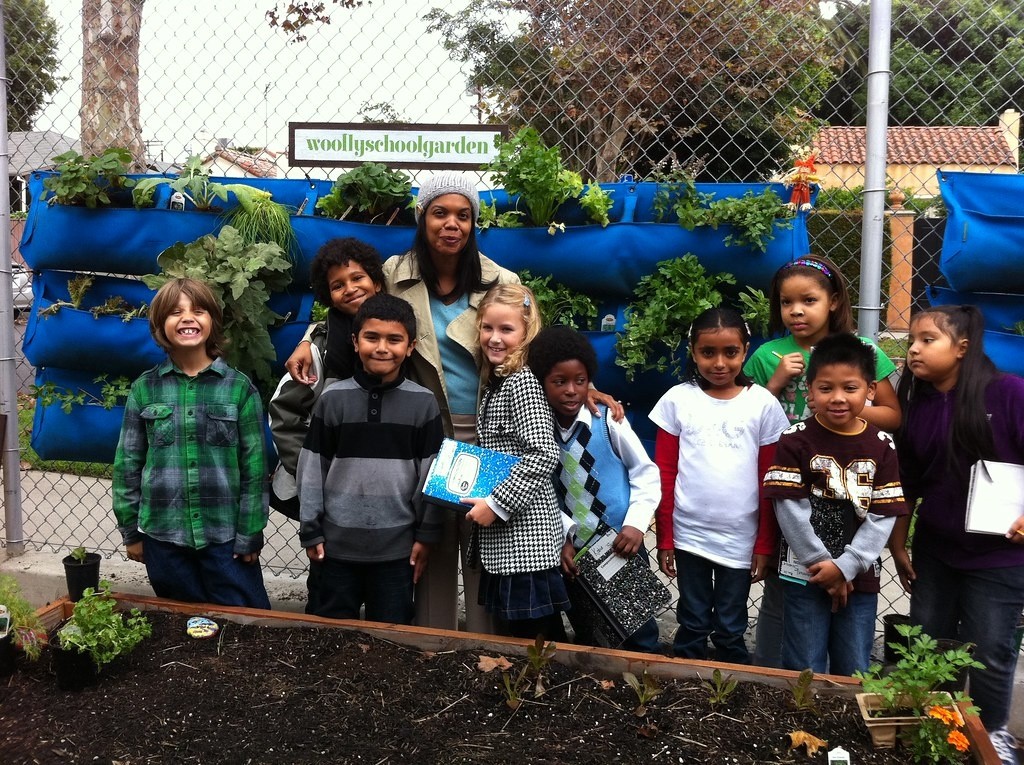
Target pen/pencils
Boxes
[771,351,808,377]
[1016,530,1024,536]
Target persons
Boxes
[889,304,1023,765]
[743,253,901,668]
[763,334,911,676]
[269,171,661,653]
[112,279,275,611]
[649,307,792,663]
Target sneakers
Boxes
[987,726,1018,765]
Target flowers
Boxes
[896,703,970,765]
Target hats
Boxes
[414,173,479,224]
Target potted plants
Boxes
[46,580,151,691]
[61,546,102,603]
[0,576,48,679]
[851,624,987,750]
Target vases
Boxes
[926,639,976,692]
[882,614,914,663]
